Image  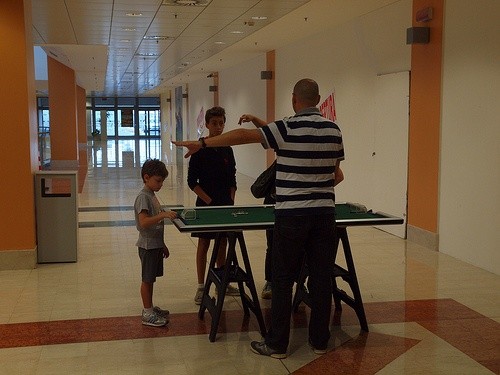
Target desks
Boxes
[163,201,404,342]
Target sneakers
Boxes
[142,306,170,317]
[141,311,170,326]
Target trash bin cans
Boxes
[35,171,78,263]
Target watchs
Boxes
[199,137,206,148]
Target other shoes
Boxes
[296,284,308,293]
[194,288,204,304]
[308,336,328,354]
[250,340,289,358]
[215,284,241,296]
[261,281,273,299]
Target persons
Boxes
[238,114,345,298]
[170,78,345,359]
[187,107,241,305]
[133,158,178,326]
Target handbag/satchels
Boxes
[250,158,277,199]
